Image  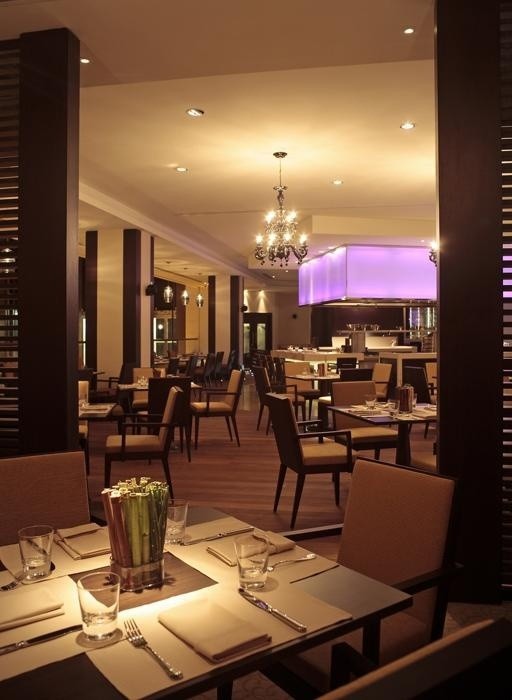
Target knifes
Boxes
[238,587,307,630]
[182,526,256,546]
[0,623,84,655]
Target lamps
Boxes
[196,292,205,307]
[251,152,309,266]
[296,243,438,308]
[163,285,173,303]
[180,290,190,306]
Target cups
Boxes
[312,368,320,377]
[364,394,377,417]
[138,375,147,386]
[388,398,400,418]
[167,499,188,543]
[233,534,270,589]
[17,525,54,577]
[76,572,123,648]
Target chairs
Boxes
[78,381,90,476]
[132,368,167,408]
[216,452,462,700]
[332,381,398,480]
[216,351,224,371]
[190,368,245,450]
[253,366,306,431]
[372,364,394,401]
[104,386,183,506]
[337,357,357,371]
[0,452,92,545]
[405,366,439,440]
[186,356,198,375]
[248,353,284,379]
[267,392,358,530]
[284,363,320,420]
[202,353,215,388]
[167,358,179,374]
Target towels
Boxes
[412,412,437,417]
[52,523,112,560]
[0,587,65,630]
[159,597,273,663]
[345,408,381,414]
[206,531,296,567]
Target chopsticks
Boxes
[101,477,169,592]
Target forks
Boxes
[0,578,23,591]
[268,553,316,572]
[122,618,182,680]
